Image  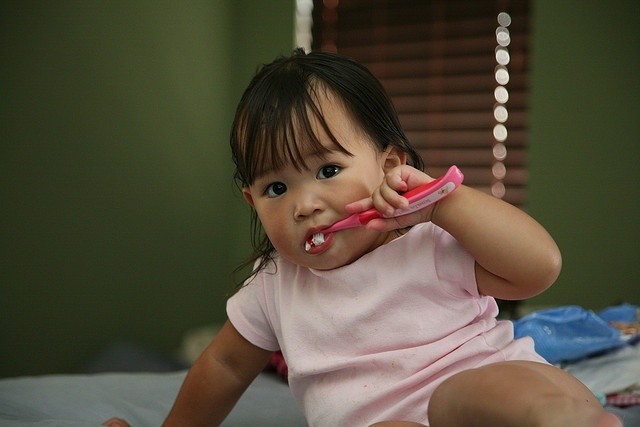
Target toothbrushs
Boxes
[304,164,464,254]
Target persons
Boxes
[101,46,623,427]
